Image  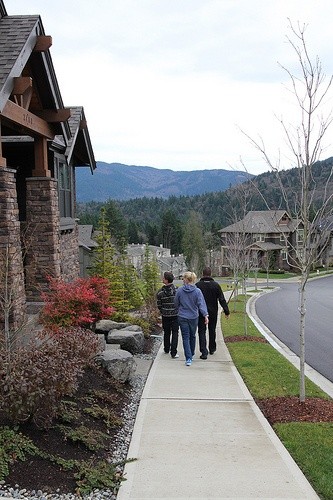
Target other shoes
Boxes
[185,362,192,366]
[200,355,207,359]
[175,354,180,358]
[210,351,215,354]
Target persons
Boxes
[155,271,180,358]
[173,271,209,366]
[194,267,230,360]
